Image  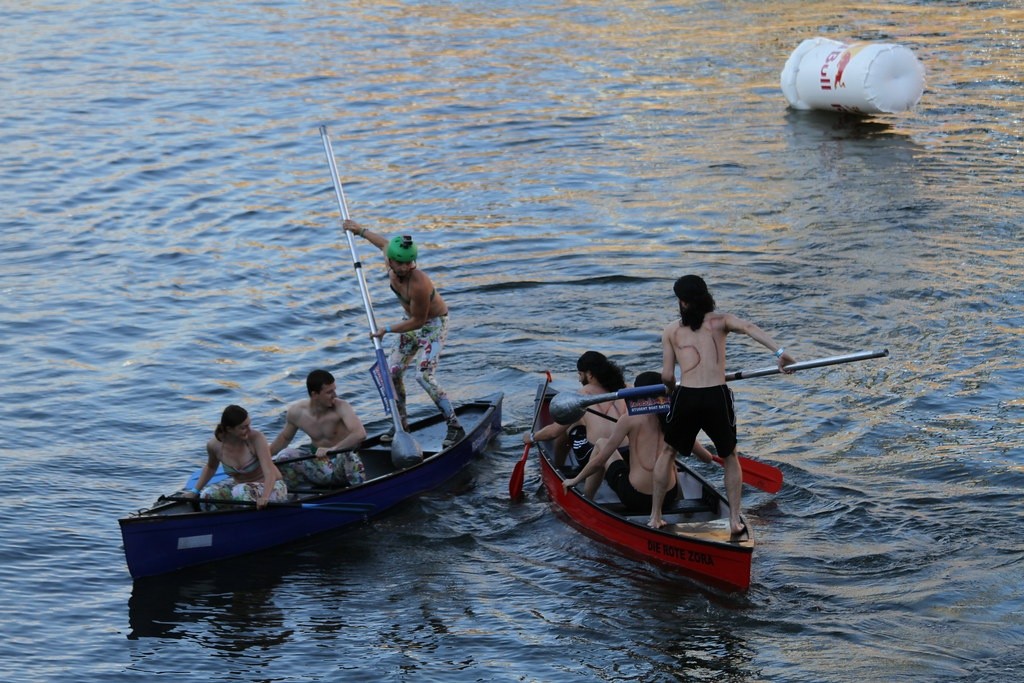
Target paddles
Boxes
[184,443,359,490]
[508,371,553,501]
[587,407,784,494]
[158,493,380,521]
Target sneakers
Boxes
[380,423,410,441]
[443,426,465,446]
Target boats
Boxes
[114,391,506,582]
[533,383,755,589]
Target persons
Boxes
[269,370,367,488]
[647,274,797,537]
[522,350,634,471]
[180,404,287,511]
[343,219,465,446]
[562,371,713,511]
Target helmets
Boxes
[387,236,417,262]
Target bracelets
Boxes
[530,433,536,444]
[193,488,201,495]
[385,325,391,333]
[775,348,783,358]
